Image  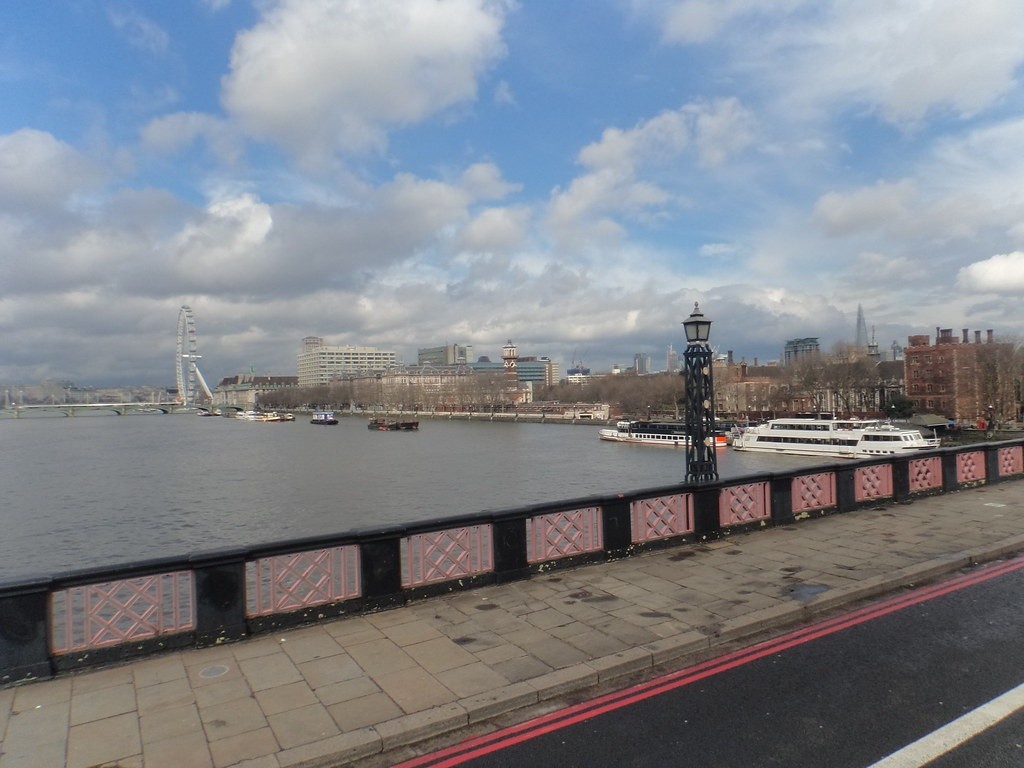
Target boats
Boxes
[196,405,296,423]
[731,418,939,459]
[365,416,420,431]
[311,412,339,425]
[598,421,729,447]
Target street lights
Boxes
[748,405,752,421]
[648,406,652,420]
[988,404,994,429]
[682,301,721,483]
[573,405,576,418]
